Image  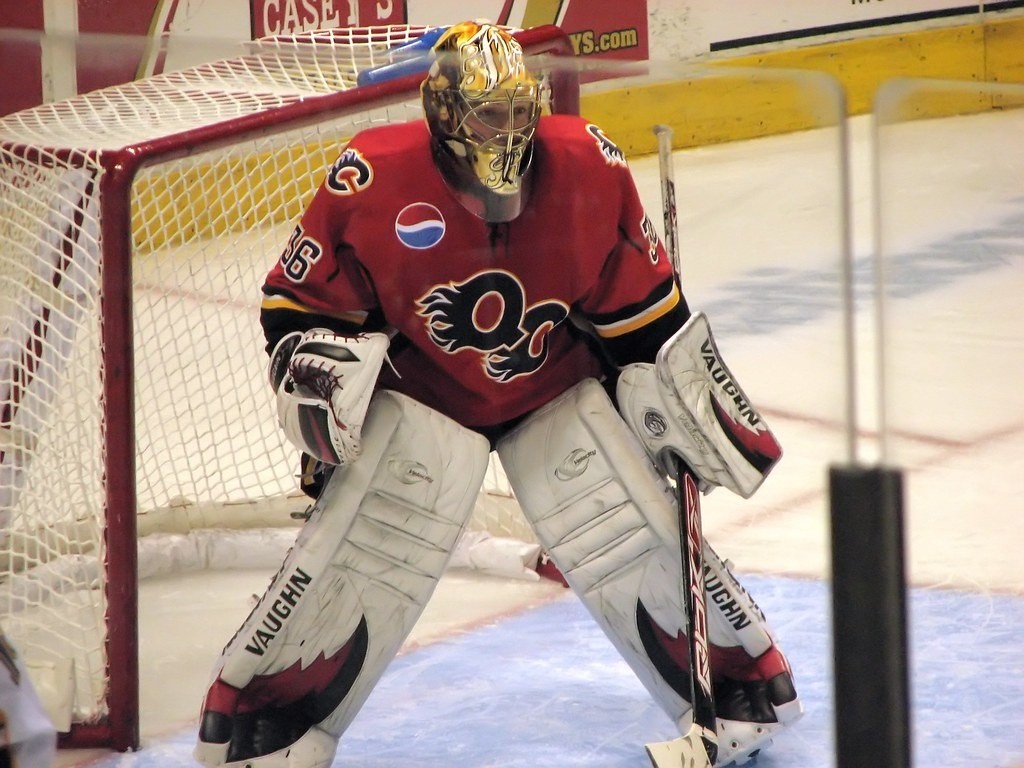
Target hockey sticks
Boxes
[642,131,722,767]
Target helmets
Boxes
[419,18,555,223]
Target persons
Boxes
[195,21,805,767]
